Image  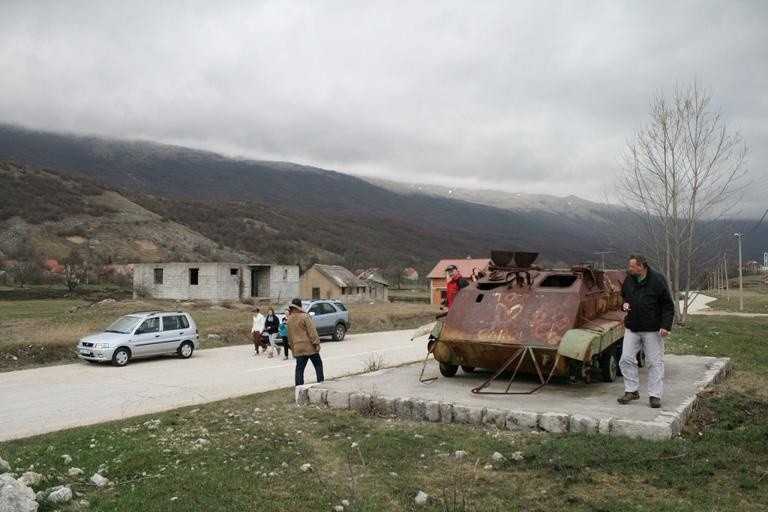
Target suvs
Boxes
[261,298,350,340]
[77,310,199,366]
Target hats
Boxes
[444,264,457,271]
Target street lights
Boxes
[707,232,743,311]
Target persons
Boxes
[249,307,267,356]
[615,253,676,409]
[278,307,292,360]
[440,264,468,311]
[265,308,282,359]
[287,297,325,386]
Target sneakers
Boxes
[268,346,281,358]
[649,397,661,408]
[617,391,640,404]
